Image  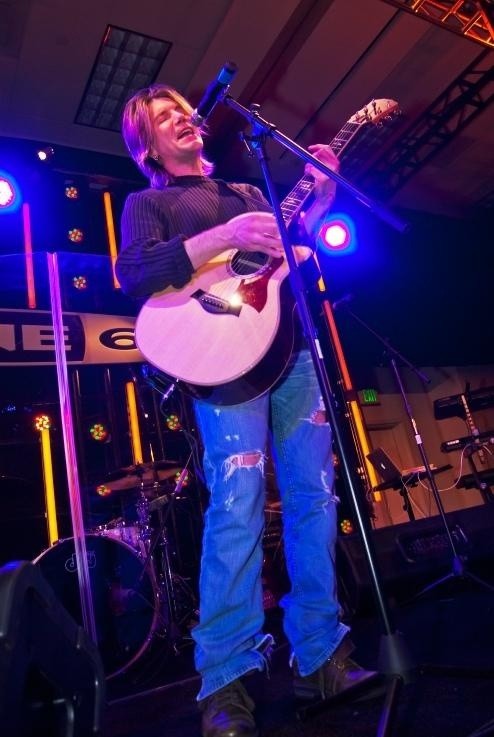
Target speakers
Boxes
[0,560,106,737]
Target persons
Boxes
[115,84,378,737]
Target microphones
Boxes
[190,61,238,127]
[319,293,353,318]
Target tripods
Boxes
[129,482,200,687]
[222,95,494,737]
[346,309,494,607]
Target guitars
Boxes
[135,98,403,405]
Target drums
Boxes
[21,536,164,693]
[96,525,143,549]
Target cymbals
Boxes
[101,462,184,492]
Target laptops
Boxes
[366,447,434,483]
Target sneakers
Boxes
[198,678,260,736]
[291,636,380,701]
